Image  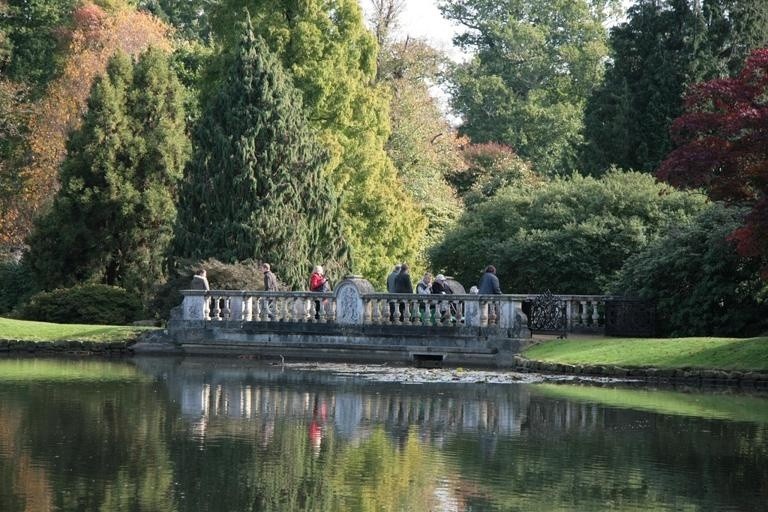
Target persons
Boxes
[310,264,329,320]
[387,265,503,322]
[262,263,278,319]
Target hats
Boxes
[434,274,447,282]
